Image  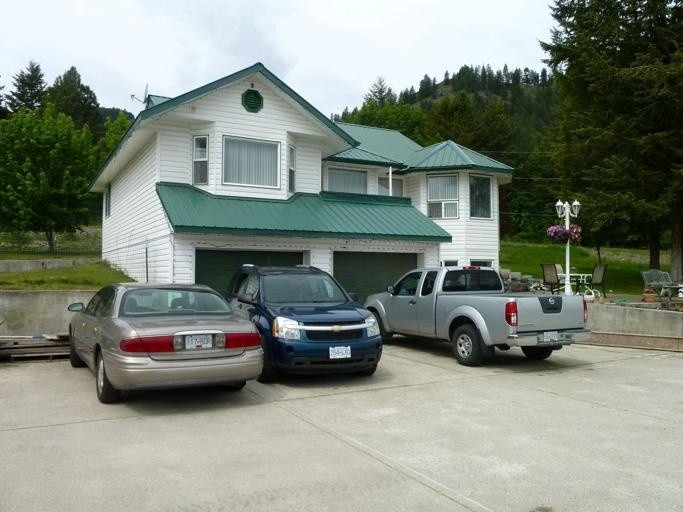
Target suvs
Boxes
[226,264,383,384]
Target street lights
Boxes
[554,199,581,295]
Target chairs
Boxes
[539,264,565,295]
[575,264,608,298]
[554,264,564,274]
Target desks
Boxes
[552,274,601,298]
[663,286,683,300]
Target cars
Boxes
[68,279,264,404]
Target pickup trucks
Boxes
[364,267,593,367]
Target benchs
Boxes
[641,269,672,297]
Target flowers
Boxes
[547,224,583,246]
